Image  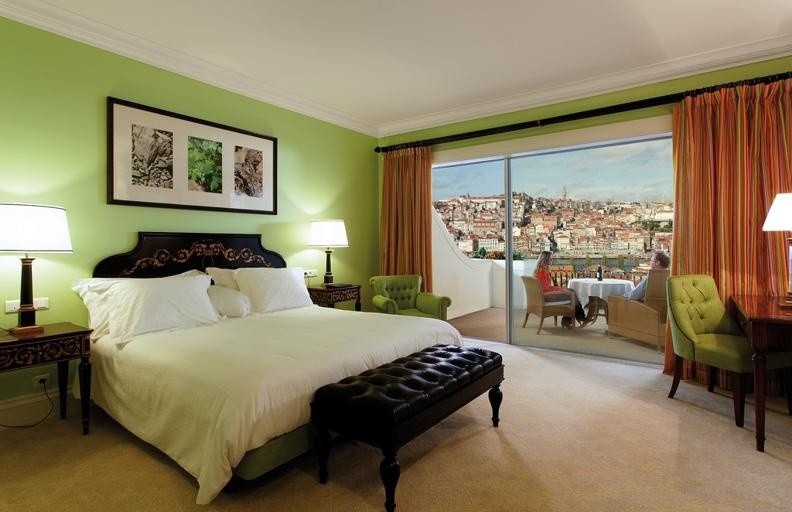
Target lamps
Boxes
[367,273,451,322]
[608,269,670,355]
[665,273,791,431]
[521,276,576,335]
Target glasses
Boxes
[759,191,792,311]
[0,202,74,337]
[302,219,349,286]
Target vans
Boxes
[561,321,574,330]
[577,317,593,324]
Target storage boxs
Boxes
[310,341,506,510]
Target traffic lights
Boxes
[73,262,314,345]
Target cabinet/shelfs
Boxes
[596,263,603,281]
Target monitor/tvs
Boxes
[777,291,792,308]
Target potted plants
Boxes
[107,95,278,217]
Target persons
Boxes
[533,251,595,329]
[606,251,671,338]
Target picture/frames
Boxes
[0,321,95,436]
[304,281,362,312]
[730,293,791,453]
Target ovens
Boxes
[71,231,460,503]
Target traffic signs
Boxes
[32,373,51,392]
[303,269,319,278]
